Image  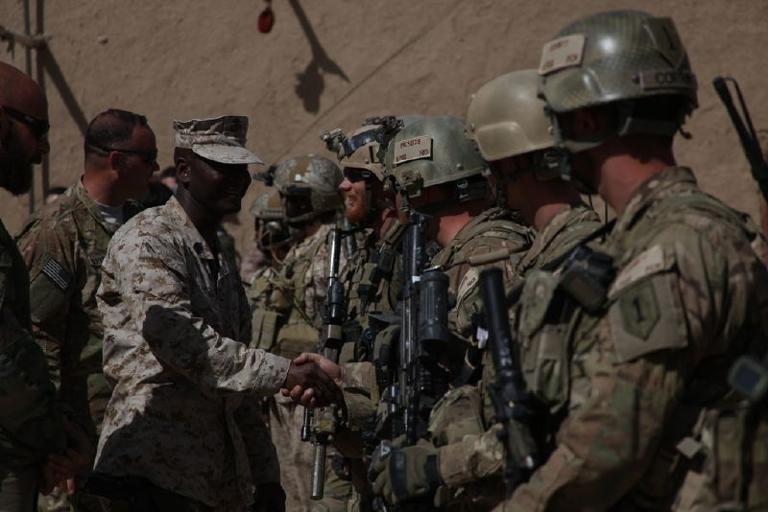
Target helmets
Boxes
[340,122,395,182]
[248,187,301,251]
[386,116,491,199]
[538,10,698,112]
[274,155,344,224]
[466,68,569,161]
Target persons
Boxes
[1,10,767,511]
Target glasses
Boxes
[1,102,50,140]
[107,147,157,163]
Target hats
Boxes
[171,116,265,166]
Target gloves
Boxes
[366,445,439,505]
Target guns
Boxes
[301,228,342,501]
[477,267,545,491]
[367,218,454,445]
